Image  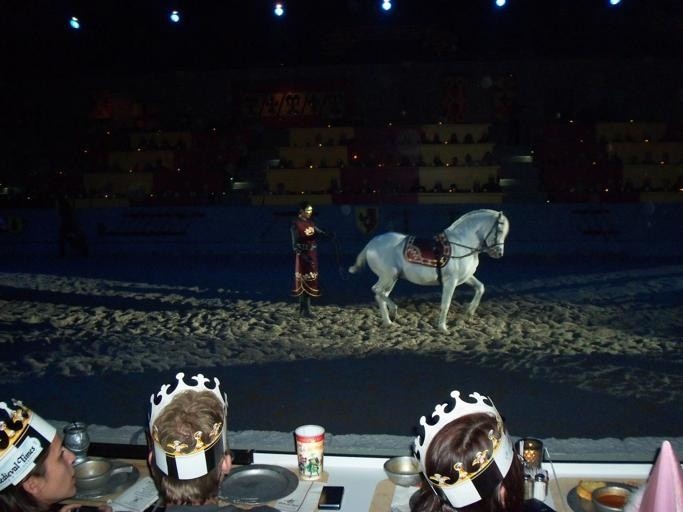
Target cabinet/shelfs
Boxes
[55,119,639,205]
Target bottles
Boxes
[524,475,533,500]
[533,474,546,502]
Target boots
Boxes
[304,297,318,320]
[299,296,305,314]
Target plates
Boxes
[71,461,140,499]
[566,482,639,512]
[218,464,298,504]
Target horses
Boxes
[348,208,510,335]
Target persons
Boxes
[288,201,335,319]
[145,371,282,512]
[0,399,112,512]
[408,389,556,511]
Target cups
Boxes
[294,424,326,481]
[63,422,90,454]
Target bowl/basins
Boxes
[591,486,633,512]
[74,458,112,489]
[384,455,421,486]
